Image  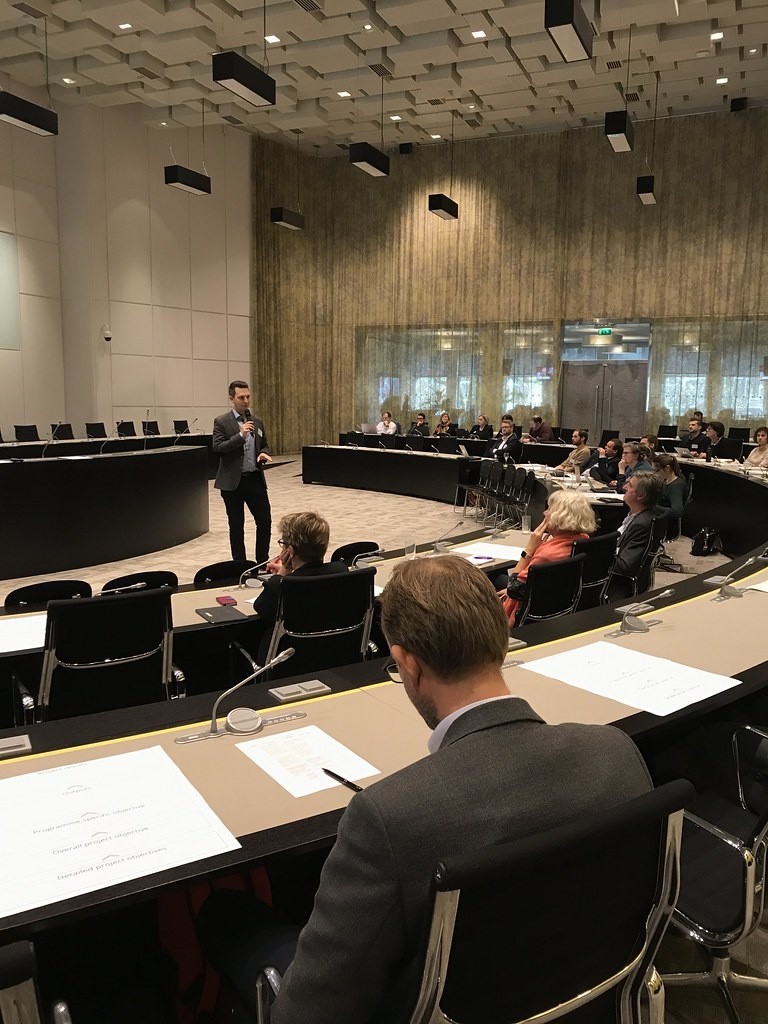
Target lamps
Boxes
[350,76,389,176]
[405,327,649,356]
[270,134,305,230]
[730,96,748,112]
[636,72,661,205]
[544,0,593,63]
[164,98,212,195]
[429,110,458,220]
[212,0,276,107]
[0,16,58,137]
[759,356,768,381]
[605,23,634,153]
[678,326,698,345]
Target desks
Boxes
[0,445,643,731]
[0,431,768,1024]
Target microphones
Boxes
[434,521,464,553]
[245,409,256,439]
[742,456,747,475]
[662,446,666,454]
[684,451,695,460]
[352,549,385,570]
[210,647,296,733]
[528,435,537,443]
[238,554,281,588]
[92,582,147,598]
[509,456,582,492]
[715,456,721,468]
[718,557,756,595]
[558,437,566,446]
[3,409,207,459]
[320,424,481,456]
[621,588,676,631]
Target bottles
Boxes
[706,448,712,462]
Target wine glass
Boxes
[503,453,510,467]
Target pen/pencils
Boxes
[322,767,363,792]
[474,556,493,559]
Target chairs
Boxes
[0,421,768,1024]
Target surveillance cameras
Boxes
[104,331,112,341]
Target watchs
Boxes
[521,551,533,560]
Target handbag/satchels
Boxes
[689,525,723,556]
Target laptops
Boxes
[674,446,705,459]
[362,423,379,434]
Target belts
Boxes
[241,472,258,476]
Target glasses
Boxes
[620,451,634,455]
[377,652,407,684]
[416,417,423,420]
[277,537,291,548]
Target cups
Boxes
[405,541,417,561]
[522,516,532,535]
[743,462,751,469]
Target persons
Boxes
[264,551,666,1024]
[555,428,686,542]
[742,426,768,469]
[406,412,430,436]
[680,418,712,454]
[489,418,520,464]
[469,414,493,440]
[254,512,351,628]
[494,413,521,439]
[694,411,710,432]
[376,412,398,435]
[494,489,598,630]
[612,469,666,597]
[433,412,458,436]
[213,380,273,569]
[520,414,554,444]
[696,421,739,462]
